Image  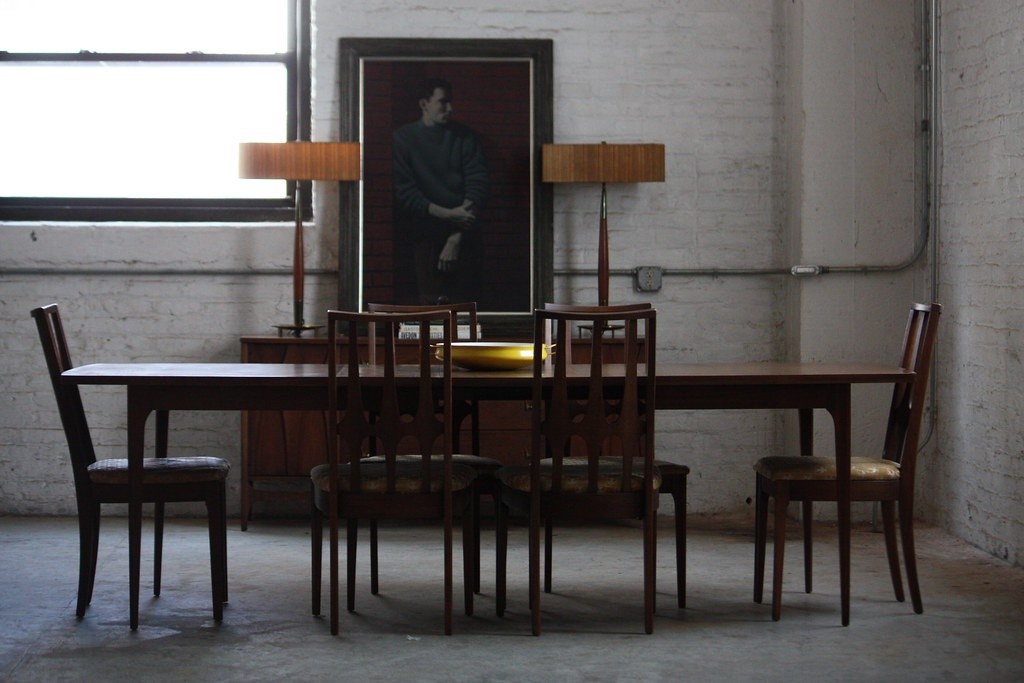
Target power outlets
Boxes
[637,265,663,291]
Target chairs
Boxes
[752,298,941,624]
[31,303,228,635]
[306,298,694,644]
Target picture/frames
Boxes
[335,38,558,331]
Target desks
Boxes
[59,363,922,644]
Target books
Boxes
[398,315,482,339]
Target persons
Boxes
[389,76,509,308]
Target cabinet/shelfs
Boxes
[238,334,665,525]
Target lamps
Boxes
[542,139,668,337]
[251,140,360,339]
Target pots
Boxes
[429,343,557,371]
